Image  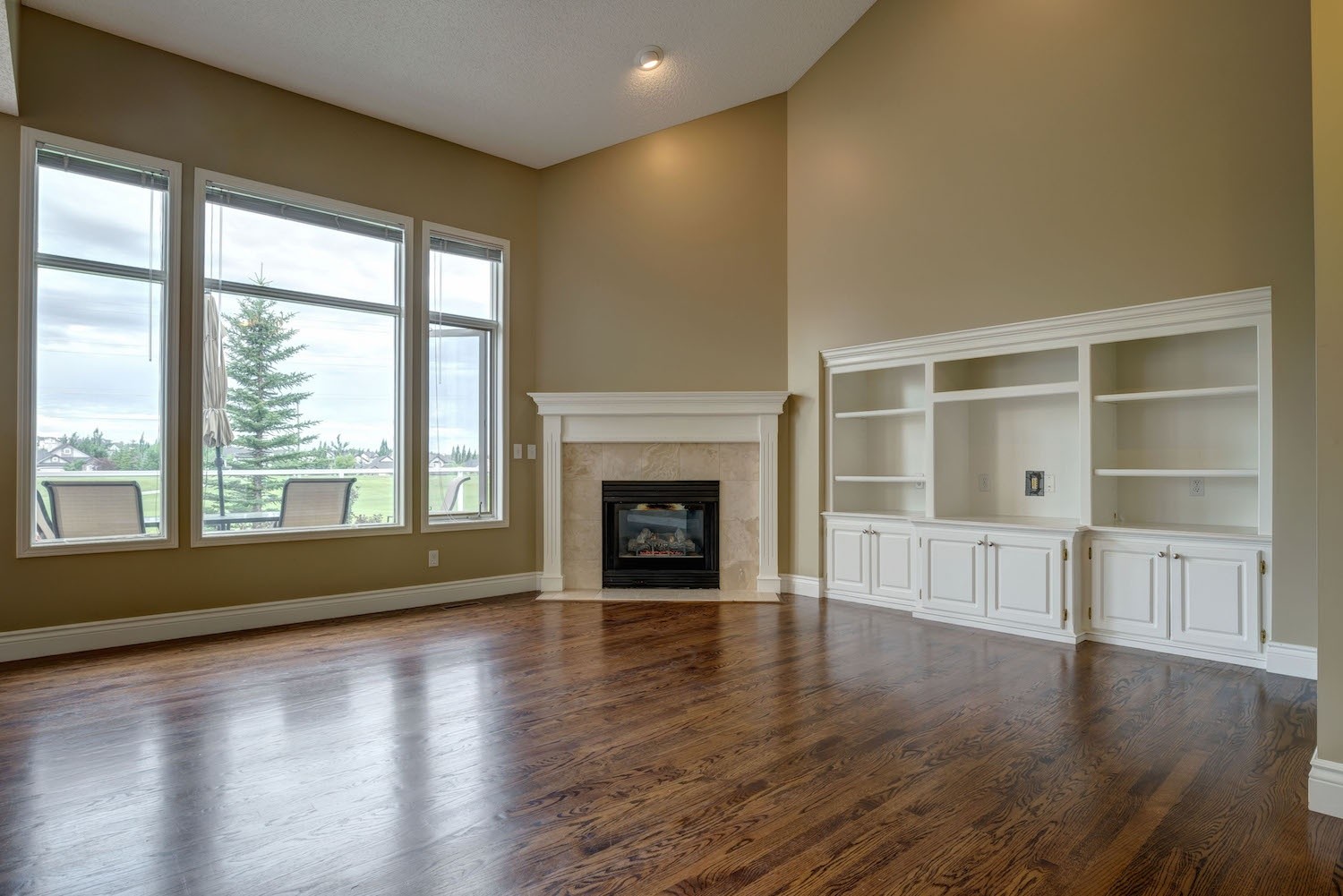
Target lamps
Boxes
[635,45,663,70]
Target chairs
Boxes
[34,476,470,543]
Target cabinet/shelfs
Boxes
[818,286,1291,668]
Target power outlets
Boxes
[429,550,439,568]
[513,444,522,459]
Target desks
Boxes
[145,512,282,534]
